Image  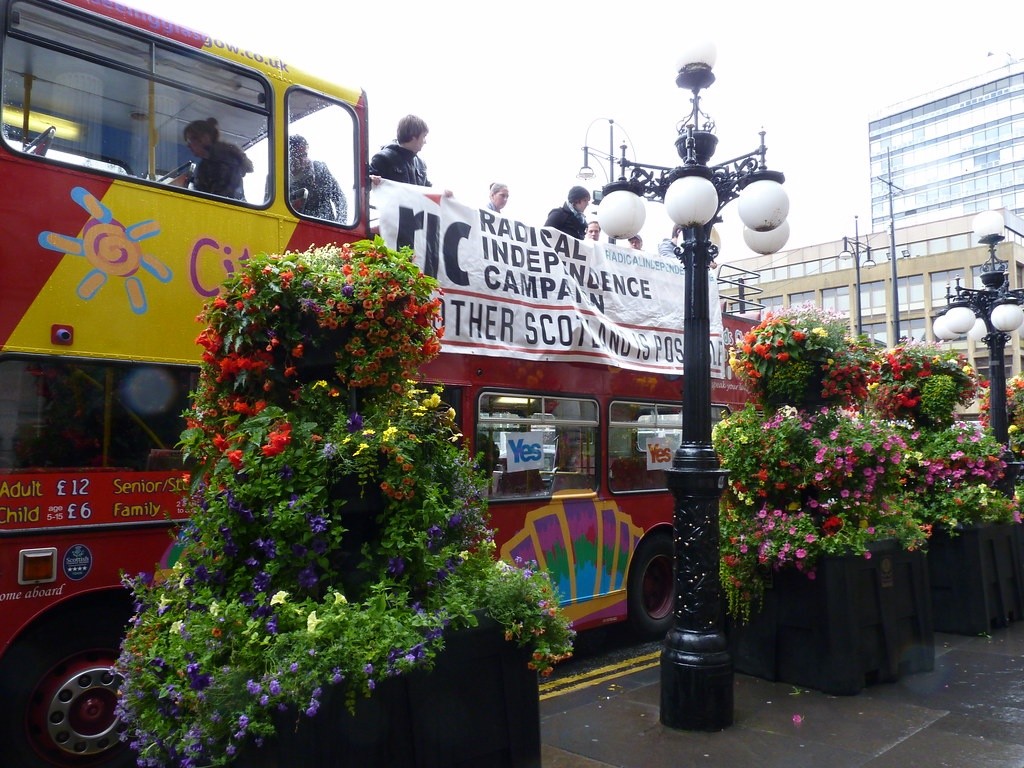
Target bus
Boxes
[0,0,766,768]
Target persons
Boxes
[184,117,253,204]
[486,182,509,214]
[629,234,644,250]
[264,134,347,224]
[544,186,602,241]
[369,114,455,198]
[659,223,718,269]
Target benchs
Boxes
[610,456,667,491]
[158,161,197,188]
[498,458,545,497]
[24,126,56,156]
[547,467,596,494]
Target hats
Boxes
[628,235,642,242]
[568,186,589,203]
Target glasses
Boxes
[185,135,195,146]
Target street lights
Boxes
[839,214,878,342]
[928,207,1024,520]
[577,42,789,732]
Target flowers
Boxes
[109,234,577,768]
[869,338,1024,537]
[979,372,1024,458]
[711,298,933,628]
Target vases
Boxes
[927,521,1024,637]
[229,606,542,768]
[724,538,935,697]
